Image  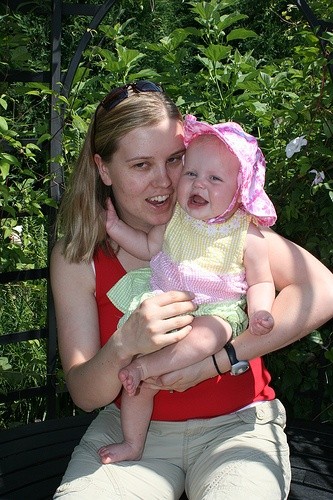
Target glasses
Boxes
[93,80,165,152]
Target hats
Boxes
[183,114,277,227]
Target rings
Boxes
[168,388,174,393]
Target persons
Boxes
[49,83,332,500]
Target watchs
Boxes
[223,341,249,376]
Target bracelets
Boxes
[211,354,222,375]
